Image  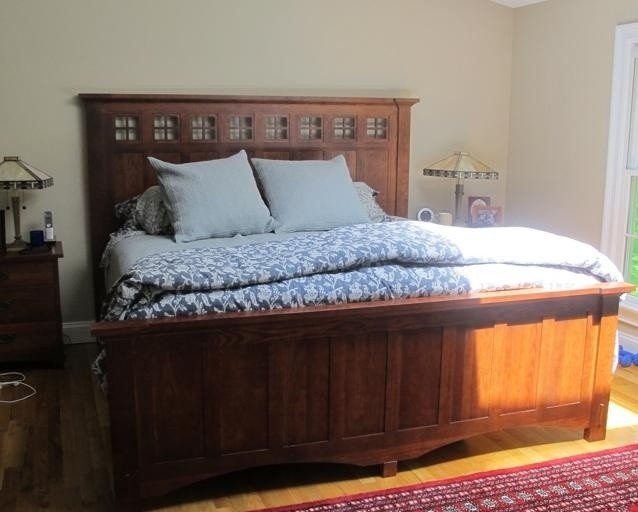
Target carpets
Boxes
[245,445,637,512]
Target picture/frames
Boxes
[466,194,503,227]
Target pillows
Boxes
[147,148,372,242]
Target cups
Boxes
[438,213,452,226]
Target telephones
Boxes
[43,211,57,243]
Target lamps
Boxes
[0,156,54,252]
[423,150,498,226]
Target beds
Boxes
[79,94,637,511]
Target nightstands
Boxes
[0,241,65,371]
